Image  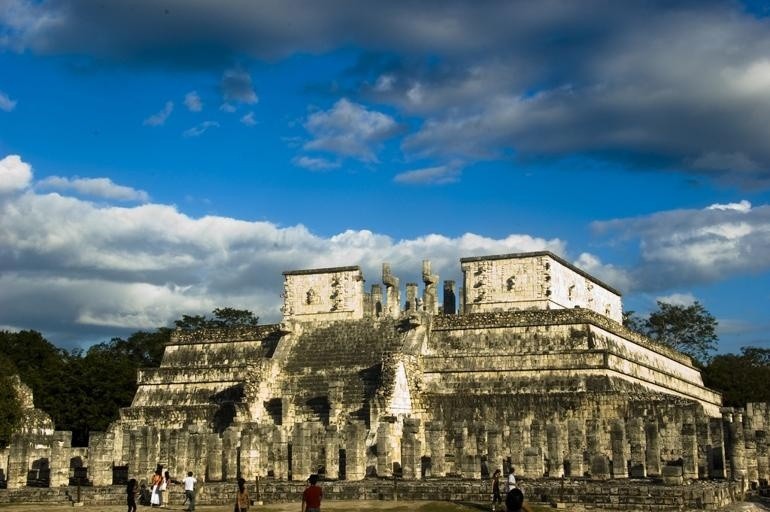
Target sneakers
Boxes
[159,504,165,508]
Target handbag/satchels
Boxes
[158,478,167,491]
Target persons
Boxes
[234,477,250,512]
[127,479,139,512]
[302,474,323,512]
[505,488,533,512]
[491,469,502,511]
[180,471,197,512]
[507,467,517,492]
[82,456,88,468]
[149,470,171,507]
